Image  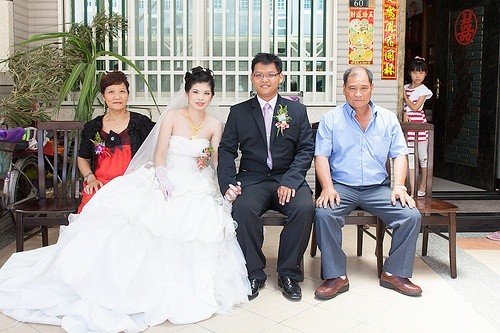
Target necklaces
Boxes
[116,125,119,127]
[187,106,205,134]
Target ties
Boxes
[263,103,273,170]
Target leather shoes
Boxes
[315,274,349,299]
[380,271,422,296]
[278,273,302,299]
[247,278,265,298]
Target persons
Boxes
[487,231,500,241]
[77,71,157,214]
[82,65,252,333]
[315,66,422,299]
[404,56,433,196]
[217,53,315,301]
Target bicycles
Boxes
[0,117,49,233]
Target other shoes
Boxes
[417,190,426,196]
[487,231,500,240]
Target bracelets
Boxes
[393,186,407,191]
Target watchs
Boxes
[84,173,93,181]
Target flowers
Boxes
[273,104,293,137]
[193,148,215,171]
[89,130,106,159]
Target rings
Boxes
[86,186,89,187]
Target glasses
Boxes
[252,70,283,80]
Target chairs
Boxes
[12,121,87,252]
[260,122,459,278]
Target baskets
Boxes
[0,141,17,180]
